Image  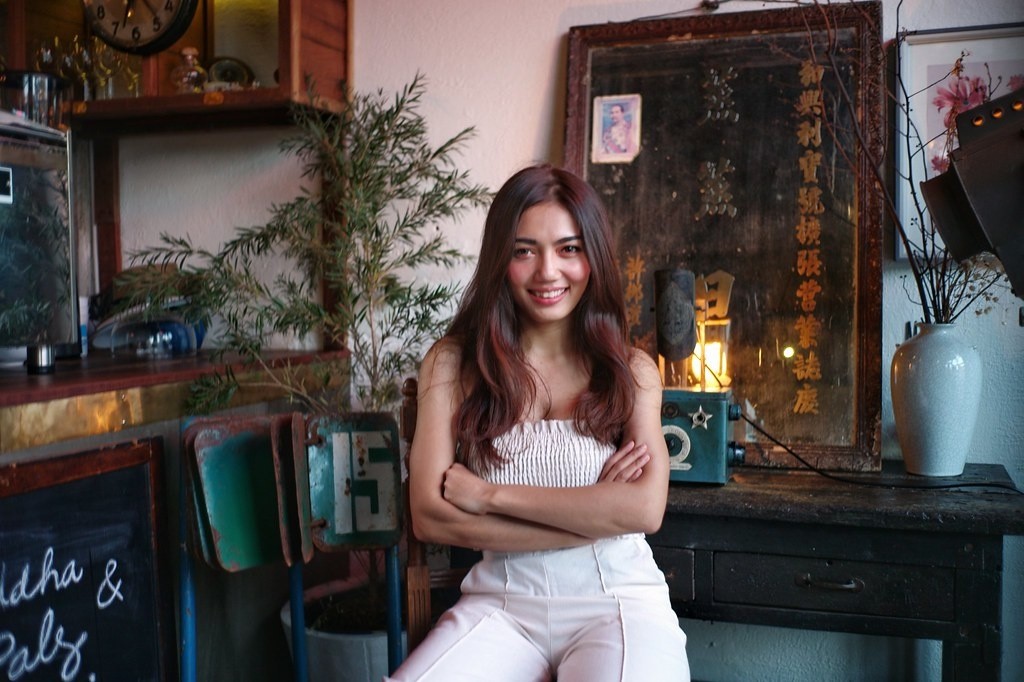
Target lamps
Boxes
[919,87,1024,324]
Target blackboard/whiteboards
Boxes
[1,438,182,682]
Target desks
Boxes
[0,347,351,682]
[645,459,1024,682]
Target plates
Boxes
[208,56,255,90]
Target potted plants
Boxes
[110,74,497,682]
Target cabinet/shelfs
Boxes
[6,0,351,137]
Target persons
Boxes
[602,104,632,154]
[383,162,693,682]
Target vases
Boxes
[890,323,984,478]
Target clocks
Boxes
[80,0,199,58]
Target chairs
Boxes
[399,377,484,659]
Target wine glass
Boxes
[35,32,142,101]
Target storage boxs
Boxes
[659,384,733,484]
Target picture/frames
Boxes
[894,21,1024,264]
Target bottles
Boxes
[170,48,206,95]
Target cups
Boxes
[19,72,57,130]
